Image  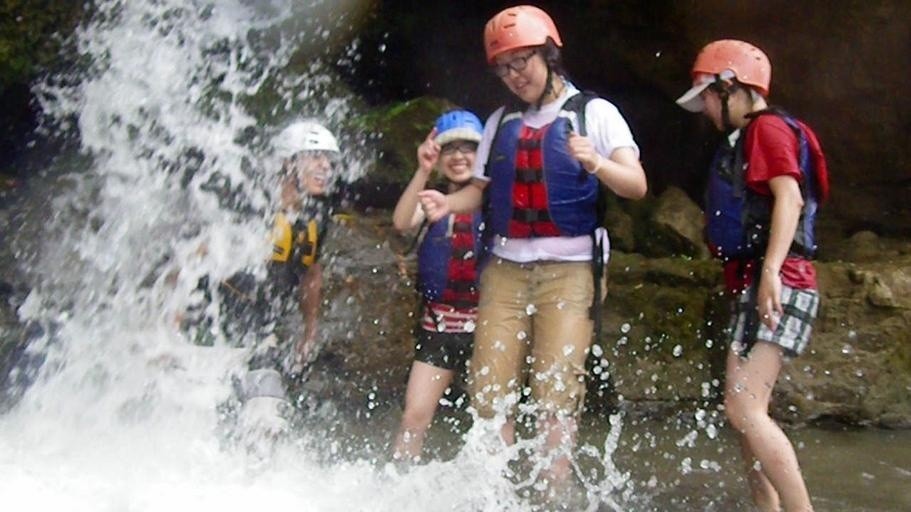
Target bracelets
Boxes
[585,155,603,175]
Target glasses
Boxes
[488,49,538,80]
[438,141,477,154]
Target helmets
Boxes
[429,107,485,147]
[481,2,564,64]
[271,121,341,176]
[673,40,773,115]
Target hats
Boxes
[673,67,735,114]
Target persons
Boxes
[673,36,831,511]
[381,110,489,474]
[417,3,647,494]
[165,119,343,489]
